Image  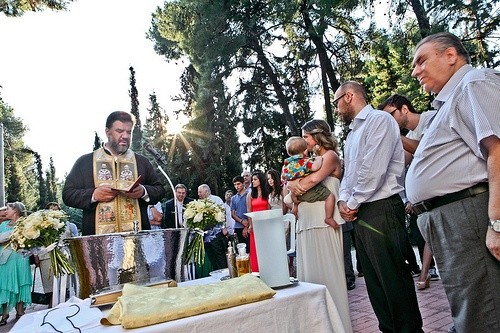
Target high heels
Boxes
[0,314,9,326]
[416,274,430,290]
[16,309,25,320]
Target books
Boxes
[90,275,176,307]
[112,175,144,193]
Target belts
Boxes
[413,181,490,216]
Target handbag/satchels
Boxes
[31,290,52,305]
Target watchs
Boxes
[489,220,500,233]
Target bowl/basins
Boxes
[209,268,233,280]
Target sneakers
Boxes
[428,267,439,281]
[411,269,421,277]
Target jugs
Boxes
[244,208,296,287]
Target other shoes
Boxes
[447,326,456,333]
[347,276,355,290]
[358,272,364,277]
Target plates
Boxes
[271,278,303,289]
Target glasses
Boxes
[332,93,344,109]
[390,108,398,116]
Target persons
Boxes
[198,184,228,270]
[162,184,194,228]
[62,111,166,237]
[147,202,163,230]
[282,136,340,230]
[224,190,239,255]
[405,32,500,333]
[332,81,425,333]
[0,201,77,325]
[330,95,457,333]
[287,121,353,333]
[231,169,296,278]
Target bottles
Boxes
[225,242,252,279]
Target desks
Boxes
[7,277,345,333]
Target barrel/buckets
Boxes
[62,228,191,300]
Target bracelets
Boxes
[241,219,244,224]
[150,206,154,208]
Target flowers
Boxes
[182,198,226,264]
[10,208,75,279]
